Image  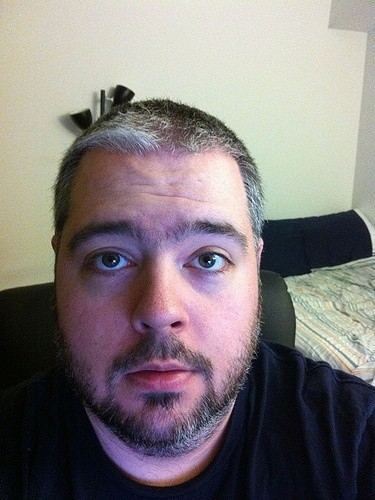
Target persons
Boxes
[0,99,375,500]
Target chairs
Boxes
[0,269,296,386]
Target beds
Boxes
[262,208,375,388]
